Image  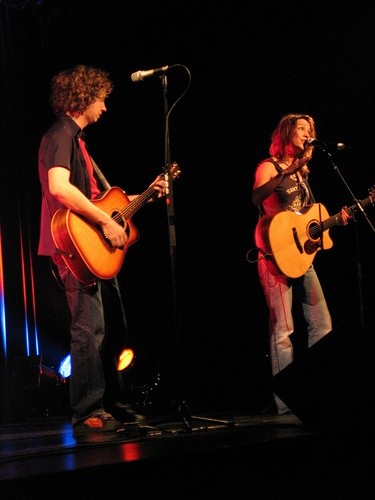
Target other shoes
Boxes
[277,404,293,415]
[117,412,146,424]
[74,409,120,432]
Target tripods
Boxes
[123,75,237,428]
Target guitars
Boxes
[49,161,183,287]
[260,188,375,282]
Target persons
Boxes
[38,66,169,434]
[251,114,353,415]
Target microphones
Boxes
[130,65,174,82]
[308,138,323,148]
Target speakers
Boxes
[267,316,375,422]
